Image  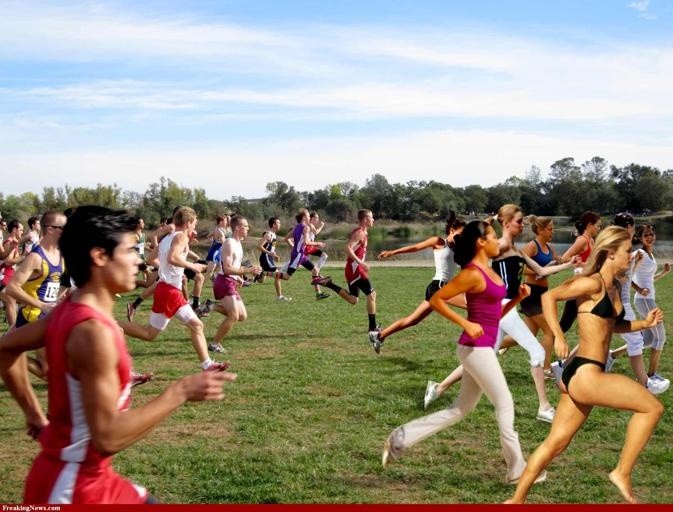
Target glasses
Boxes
[544,369,557,380]
[44,224,64,230]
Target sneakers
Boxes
[241,256,251,267]
[550,360,564,385]
[126,302,135,322]
[423,380,440,411]
[368,331,384,354]
[376,326,384,332]
[202,360,230,371]
[644,375,670,393]
[507,469,546,484]
[606,350,613,373]
[253,270,263,283]
[311,275,331,287]
[198,298,211,318]
[207,343,225,353]
[193,303,209,317]
[650,372,667,381]
[535,406,556,423]
[316,292,330,300]
[130,371,153,387]
[278,296,292,301]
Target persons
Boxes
[135,217,175,289]
[253,208,331,300]
[368,210,467,353]
[549,212,669,394]
[303,211,329,272]
[423,204,582,424]
[197,214,263,353]
[126,205,210,321]
[19,217,41,262]
[0,220,7,243]
[0,219,26,327]
[0,204,239,504]
[117,207,231,373]
[501,225,665,505]
[134,217,152,282]
[381,220,548,486]
[0,238,19,259]
[241,217,288,300]
[559,212,603,333]
[5,211,69,382]
[499,214,564,381]
[205,214,227,279]
[311,208,382,332]
[604,223,671,385]
